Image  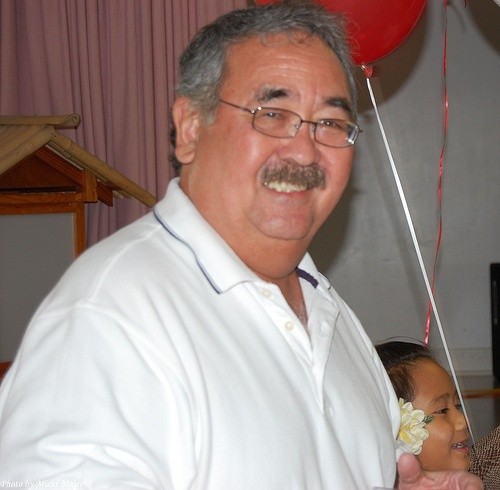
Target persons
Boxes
[374,341,485,490]
[0,0,403,490]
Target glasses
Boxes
[210,94,363,149]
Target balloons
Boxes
[316,0,426,78]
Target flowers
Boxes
[397,397,436,465]
